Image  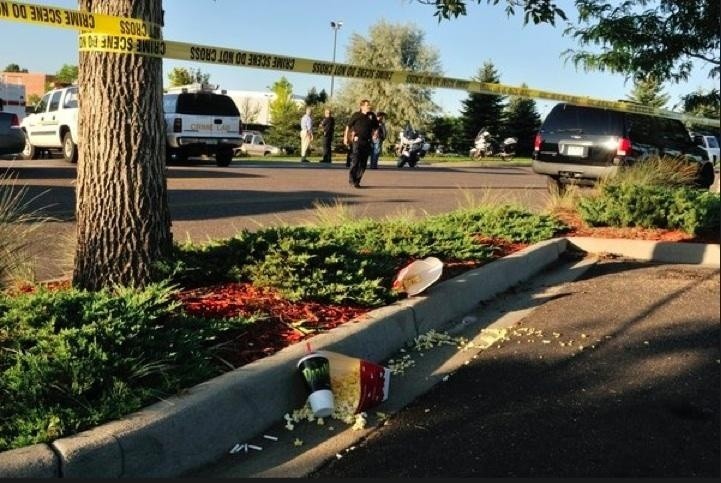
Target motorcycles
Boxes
[468,126,515,160]
[395,127,430,168]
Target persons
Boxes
[398,119,424,166]
[343,99,379,188]
[370,112,387,169]
[299,107,314,163]
[317,109,334,163]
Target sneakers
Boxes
[302,156,331,163]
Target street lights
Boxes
[328,20,342,100]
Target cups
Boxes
[296,352,335,418]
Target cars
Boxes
[0,81,290,166]
[531,94,720,199]
